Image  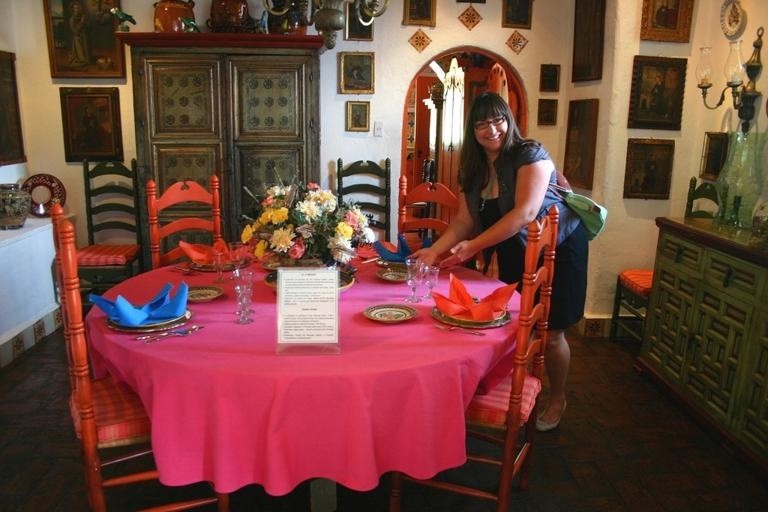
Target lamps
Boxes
[263,0,389,49]
[694,26,764,143]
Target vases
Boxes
[152,0,195,32]
[205,0,256,34]
[0,184,32,230]
[267,0,307,34]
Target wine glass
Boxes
[233,267,256,325]
[210,242,247,282]
[402,258,439,302]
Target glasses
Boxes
[473,115,505,129]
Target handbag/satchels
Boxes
[560,188,607,240]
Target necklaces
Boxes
[479,161,498,211]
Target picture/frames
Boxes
[400,1,440,29]
[561,97,601,194]
[621,137,678,200]
[337,49,378,96]
[43,0,126,78]
[534,97,560,127]
[639,2,698,45]
[569,0,607,87]
[344,100,372,134]
[499,1,534,31]
[624,53,690,134]
[59,87,124,162]
[0,50,27,167]
[537,61,564,95]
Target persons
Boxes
[640,152,658,193]
[655,0,670,28]
[408,95,590,431]
[354,111,362,127]
[649,73,666,119]
[68,2,90,66]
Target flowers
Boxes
[237,178,381,286]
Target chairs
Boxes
[332,157,394,245]
[610,177,742,343]
[53,201,232,511]
[142,174,225,272]
[406,206,560,511]
[392,172,469,270]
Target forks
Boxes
[145,324,200,343]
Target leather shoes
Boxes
[536,399,567,431]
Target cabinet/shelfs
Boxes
[114,31,323,272]
[633,217,768,483]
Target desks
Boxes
[0,210,80,369]
[84,236,538,511]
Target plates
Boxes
[363,299,512,329]
[375,257,419,283]
[105,286,224,332]
[188,260,250,272]
